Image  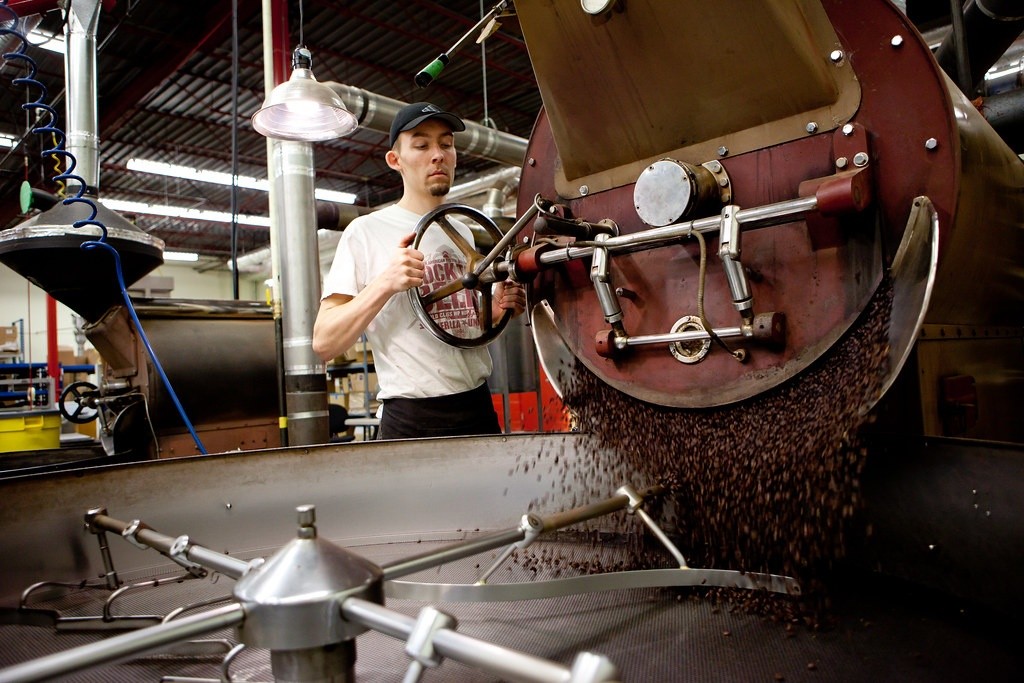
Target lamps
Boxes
[249,0,358,142]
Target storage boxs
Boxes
[326,342,380,409]
[0,325,19,352]
[57,345,101,398]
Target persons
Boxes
[312,101,526,439]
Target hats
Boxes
[388,101,465,150]
